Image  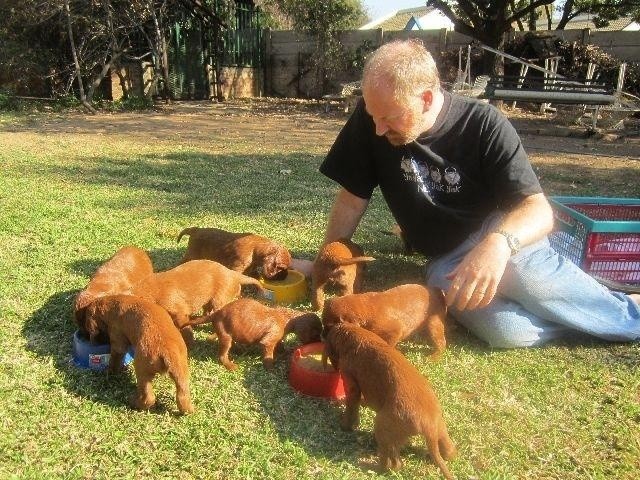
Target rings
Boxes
[473,288,486,296]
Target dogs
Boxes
[178,297,322,376]
[71,244,155,332]
[83,292,197,416]
[321,282,450,363]
[178,226,295,284]
[135,257,274,350]
[321,323,459,480]
[309,236,378,315]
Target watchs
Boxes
[489,227,521,255]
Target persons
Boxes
[288,38,640,350]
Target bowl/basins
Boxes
[286,341,346,398]
[255,269,309,303]
[71,327,134,371]
